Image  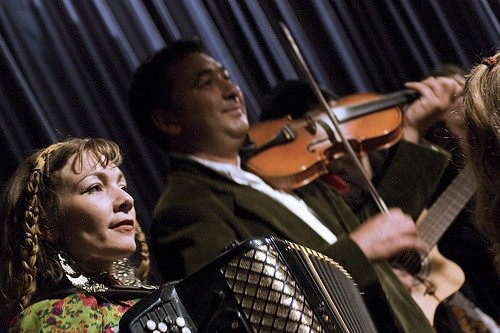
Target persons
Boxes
[126,36,434,333]
[0,138,159,333]
[461,52,500,275]
[258,62,467,215]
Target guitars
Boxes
[387,163,477,328]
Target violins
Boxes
[238,90,420,190]
